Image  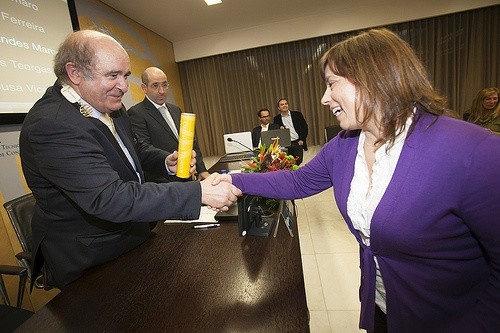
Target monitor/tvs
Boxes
[238,194,275,236]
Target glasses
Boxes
[147,83,170,89]
[260,115,271,118]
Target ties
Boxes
[160,107,178,138]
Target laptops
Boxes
[224,131,253,155]
[261,128,291,148]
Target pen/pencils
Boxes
[191,223,221,229]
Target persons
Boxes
[275,99,309,166]
[251,109,279,148]
[128,66,210,182]
[208,29,500,333]
[19,29,242,294]
[467,86,500,134]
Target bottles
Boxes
[220,170,227,174]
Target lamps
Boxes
[205,0,222,6]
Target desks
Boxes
[11,152,310,333]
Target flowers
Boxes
[239,135,299,209]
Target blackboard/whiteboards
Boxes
[0,124,31,202]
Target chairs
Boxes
[3,193,45,289]
[0,265,36,333]
[324,127,342,144]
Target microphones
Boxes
[228,138,254,152]
